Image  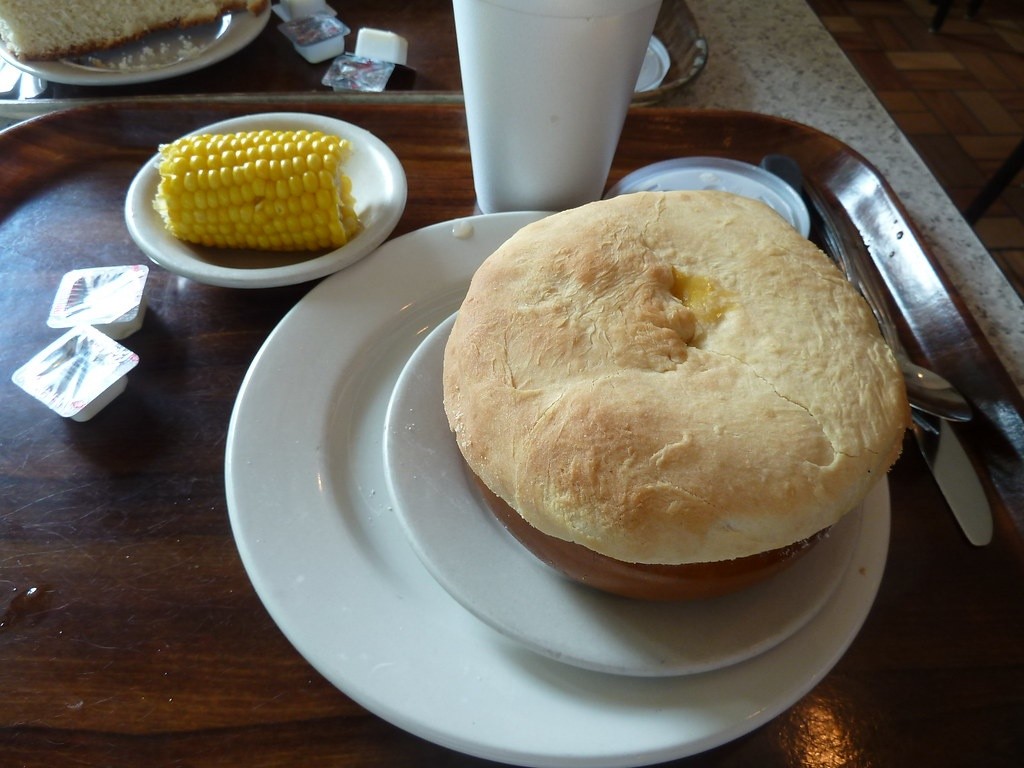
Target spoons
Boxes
[803,174,973,423]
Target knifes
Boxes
[801,177,994,547]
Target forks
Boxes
[758,155,940,437]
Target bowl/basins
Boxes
[123,113,409,289]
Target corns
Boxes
[151,130,363,248]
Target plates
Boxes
[1,0,273,87]
[384,312,859,679]
[222,211,892,768]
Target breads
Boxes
[442,190,913,603]
[0,1,267,61]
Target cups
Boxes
[453,0,664,213]
